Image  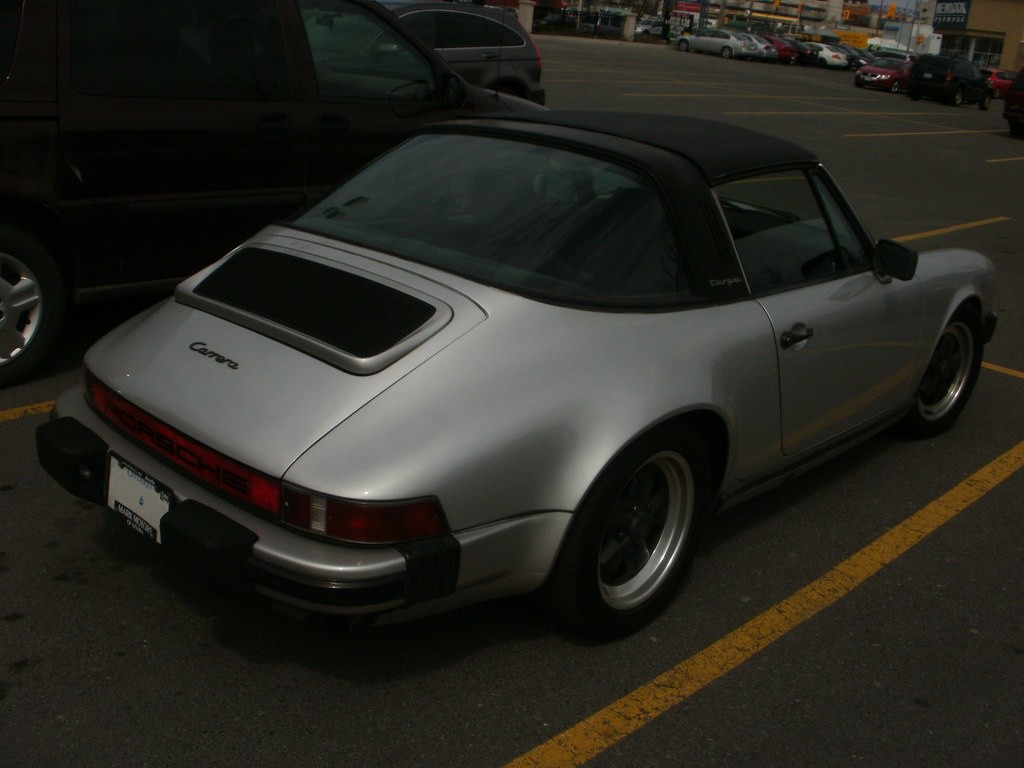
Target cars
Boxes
[979,67,1017,99]
[855,58,913,93]
[635,19,670,35]
[1003,65,1024,137]
[301,0,545,105]
[676,28,759,58]
[0,0,552,384]
[907,53,991,109]
[738,33,779,63]
[762,35,800,63]
[803,42,851,68]
[834,44,920,66]
[784,38,819,65]
[36,111,998,641]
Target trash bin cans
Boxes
[485,4,519,20]
